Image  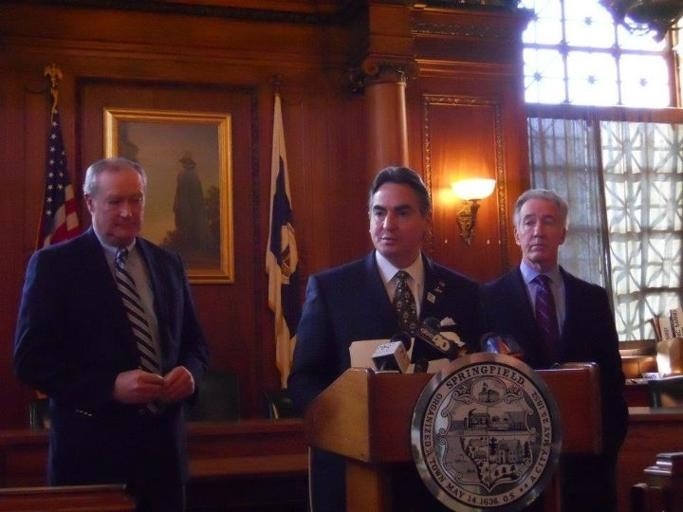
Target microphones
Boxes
[481,332,521,361]
[422,317,474,362]
[372,331,412,374]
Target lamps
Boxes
[447,177,498,245]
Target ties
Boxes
[534,275,559,361]
[114,248,159,414]
[393,271,418,337]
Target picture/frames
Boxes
[103,106,237,288]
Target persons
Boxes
[285,165,482,512]
[479,187,631,510]
[12,156,210,511]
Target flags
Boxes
[266,90,303,394]
[34,67,83,430]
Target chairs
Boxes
[25,368,301,427]
[647,375,683,410]
